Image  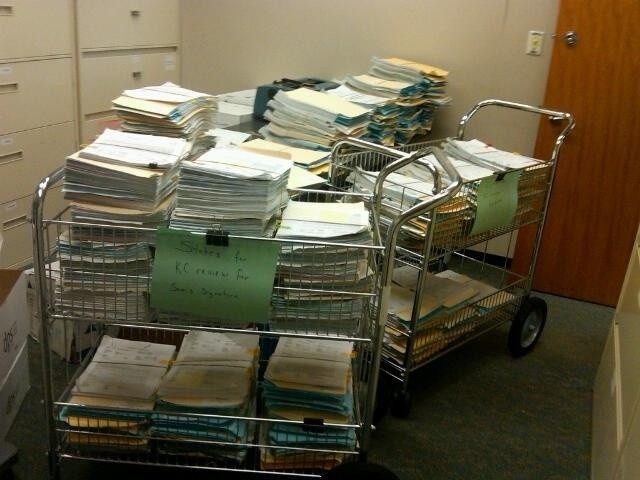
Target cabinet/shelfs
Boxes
[590,227,640,480]
[1,1,182,274]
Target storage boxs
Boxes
[1,251,129,445]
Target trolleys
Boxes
[322,96,577,418]
[34,132,460,480]
